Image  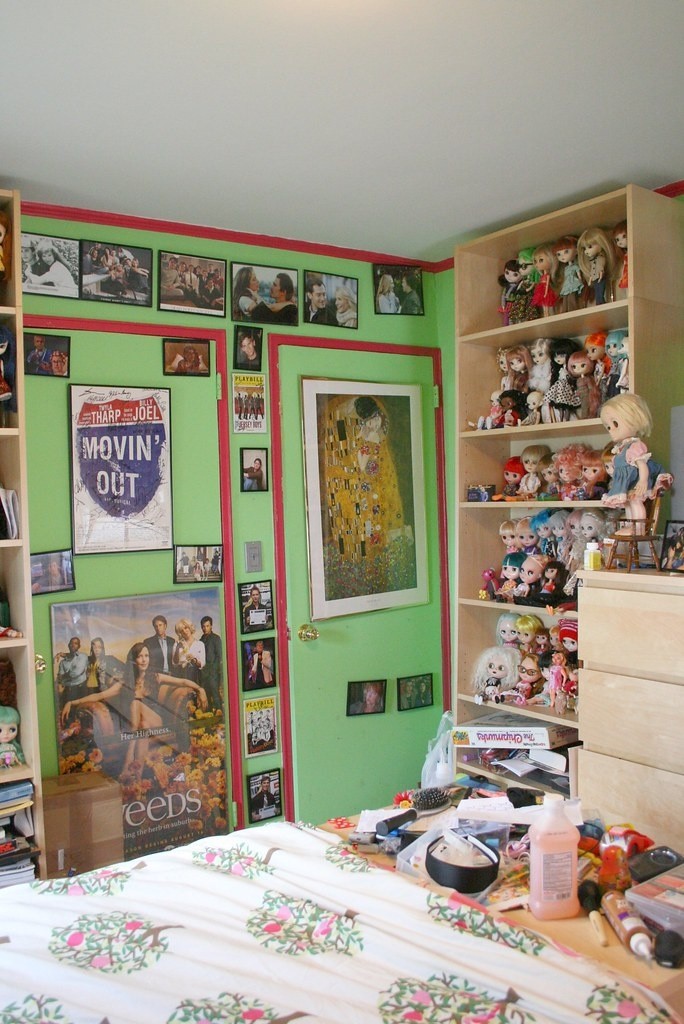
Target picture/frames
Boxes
[162,338,211,378]
[241,637,277,692]
[660,520,684,573]
[82,239,153,307]
[372,263,424,315]
[246,768,282,824]
[346,678,387,715]
[22,231,81,299]
[300,379,430,623]
[49,586,230,862]
[233,325,263,372]
[397,672,437,712]
[23,332,70,378]
[173,544,223,584]
[304,269,359,330]
[30,548,75,596]
[240,447,269,492]
[230,261,299,326]
[158,250,228,317]
[237,579,275,634]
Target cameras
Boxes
[626,844,684,882]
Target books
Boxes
[0,780,41,890]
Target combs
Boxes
[376,788,453,835]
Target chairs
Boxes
[604,496,661,573]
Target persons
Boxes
[350,682,383,714]
[177,549,221,581]
[376,270,423,314]
[243,458,263,490]
[476,329,629,430]
[0,211,12,305]
[491,440,617,502]
[497,507,634,616]
[470,612,578,708]
[663,527,684,571]
[21,238,79,289]
[53,613,224,787]
[0,657,26,768]
[32,554,72,593]
[251,776,281,821]
[497,220,628,327]
[245,586,275,689]
[175,347,201,373]
[238,330,264,420]
[82,242,356,328]
[601,393,673,536]
[27,336,67,376]
[401,676,431,710]
[0,326,17,426]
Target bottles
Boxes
[529,793,580,919]
[601,891,653,963]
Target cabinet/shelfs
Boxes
[575,568,684,838]
[453,182,684,801]
[0,187,48,880]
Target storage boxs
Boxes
[42,770,122,880]
